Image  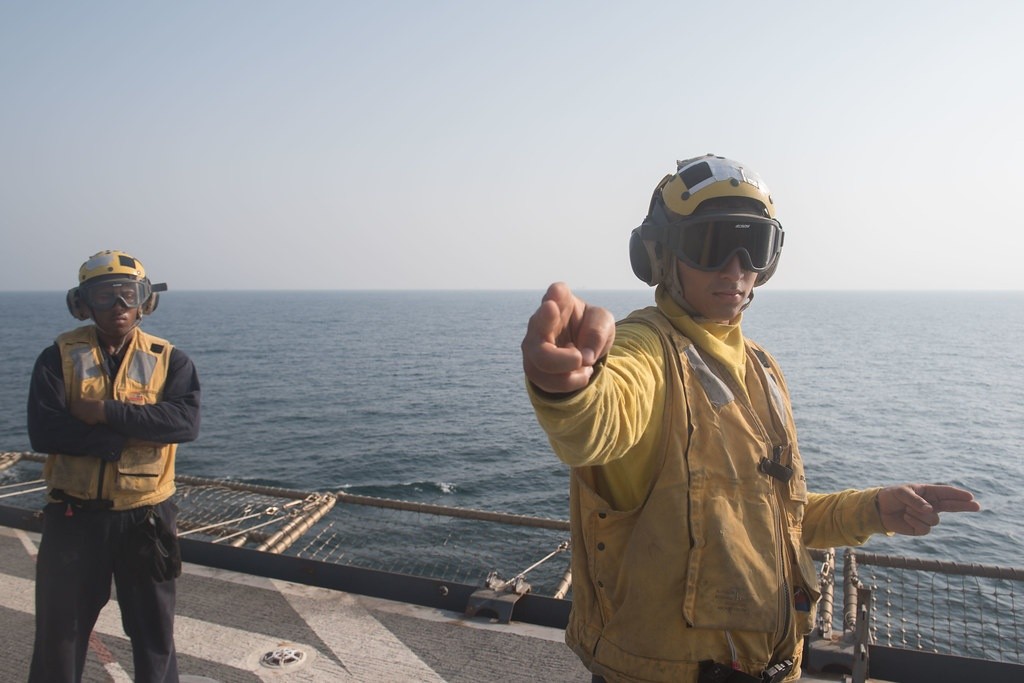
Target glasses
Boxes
[640,213,784,272]
[77,279,152,311]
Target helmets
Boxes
[629,153,786,287]
[66,249,159,321]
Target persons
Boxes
[522,154,979,683]
[27,250,200,683]
